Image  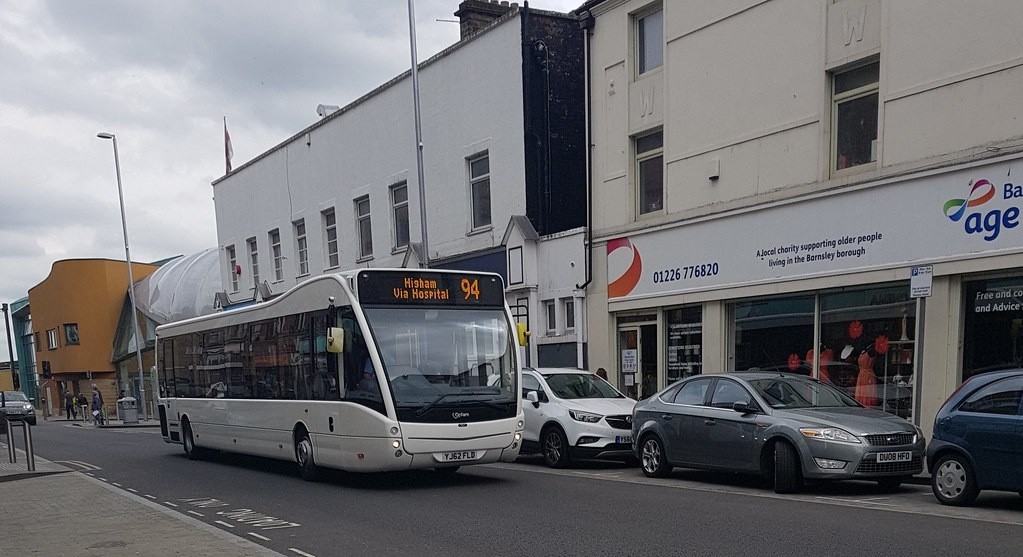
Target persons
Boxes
[118,390,125,399]
[854,333,878,407]
[806,334,833,386]
[91,384,105,425]
[91,390,102,426]
[64,390,89,420]
[363,336,395,379]
[590,368,612,398]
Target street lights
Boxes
[97,132,150,421]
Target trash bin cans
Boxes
[121,397,139,425]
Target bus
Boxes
[153,265,533,482]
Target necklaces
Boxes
[820,351,826,358]
[861,343,873,353]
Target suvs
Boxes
[486,366,638,468]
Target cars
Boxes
[925,369,1023,505]
[630,370,925,495]
[0,391,37,427]
